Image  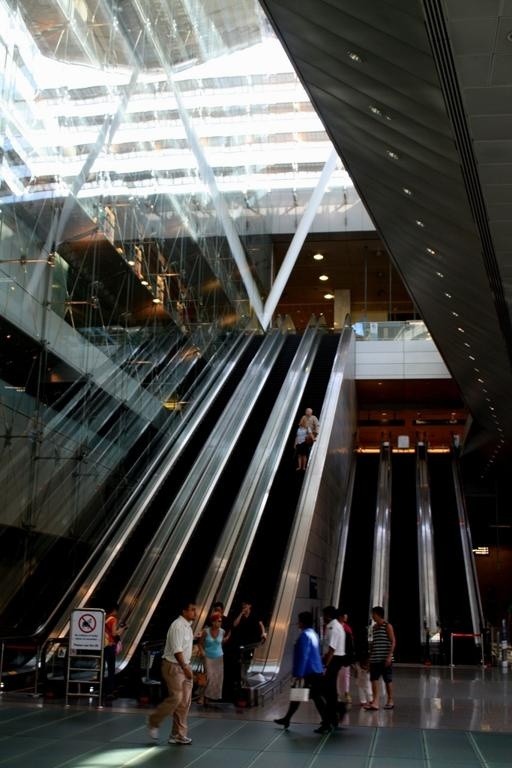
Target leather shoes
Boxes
[314,724,332,734]
[274,716,289,729]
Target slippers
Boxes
[384,702,394,709]
[362,702,379,710]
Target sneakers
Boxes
[167,732,192,744]
[145,714,161,740]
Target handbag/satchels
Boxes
[288,677,309,702]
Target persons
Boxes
[320,605,396,729]
[293,415,316,472]
[147,602,198,747]
[299,406,320,438]
[194,600,268,712]
[104,602,130,698]
[272,611,336,738]
